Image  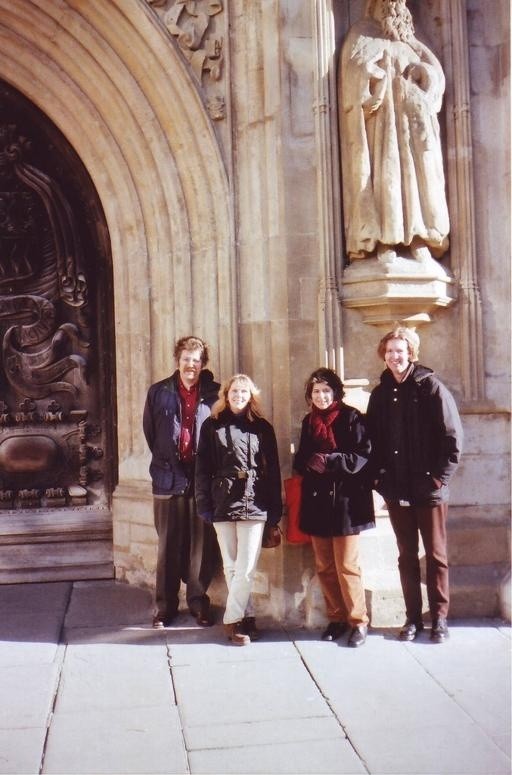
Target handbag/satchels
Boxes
[284,468,314,544]
[262,525,281,548]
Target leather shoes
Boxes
[322,622,347,641]
[242,617,259,640]
[431,619,449,643]
[223,623,251,645]
[400,620,424,641]
[348,626,367,647]
[152,614,170,628]
[191,610,213,626]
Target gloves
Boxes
[200,511,213,525]
[267,512,281,526]
[308,453,327,474]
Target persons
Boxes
[143,335,225,628]
[291,367,377,647]
[195,375,283,644]
[338,0,453,265]
[367,330,463,644]
